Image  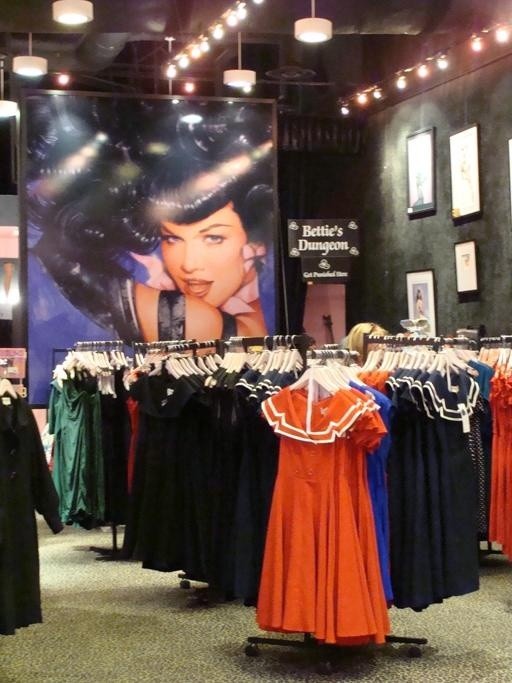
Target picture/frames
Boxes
[406,126,436,217]
[405,123,512,337]
[449,123,480,219]
[455,239,479,293]
[405,269,436,335]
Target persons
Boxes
[26,94,276,354]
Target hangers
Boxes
[0,332,512,401]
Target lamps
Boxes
[52,1,95,25]
[437,56,450,69]
[342,106,350,114]
[396,75,407,90]
[13,56,49,78]
[357,93,369,107]
[294,18,333,42]
[240,85,254,94]
[223,71,256,88]
[470,36,486,52]
[419,64,432,79]
[165,0,262,78]
[56,73,70,85]
[1,100,20,117]
[495,26,511,43]
[373,90,383,99]
[183,82,196,94]
